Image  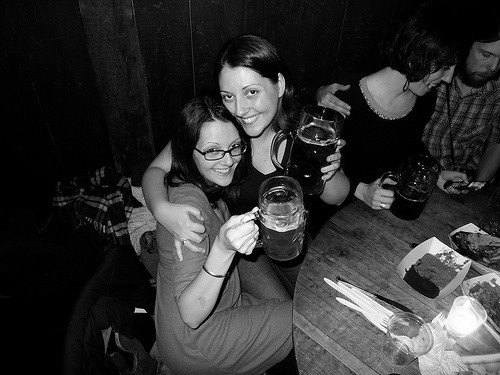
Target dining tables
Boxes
[292,186,500,375]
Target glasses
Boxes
[194,139,249,161]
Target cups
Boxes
[381,312,433,370]
[378,154,442,221]
[445,296,488,338]
[240,175,305,261]
[270,105,345,195]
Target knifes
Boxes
[335,275,415,315]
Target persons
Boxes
[314,28,500,182]
[154,97,293,375]
[141,35,351,304]
[325,10,470,210]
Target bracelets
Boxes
[203,264,226,278]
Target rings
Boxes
[182,240,190,245]
[380,202,386,208]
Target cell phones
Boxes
[444,180,490,193]
